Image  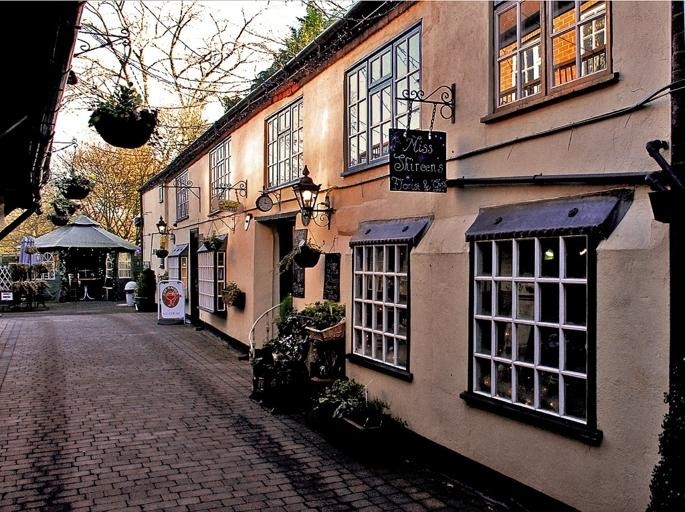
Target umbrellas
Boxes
[30,215,141,300]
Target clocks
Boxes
[256,195,273,212]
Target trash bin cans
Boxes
[125,281,138,307]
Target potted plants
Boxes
[269,235,326,276]
[297,300,346,342]
[87,82,163,149]
[152,245,169,258]
[43,163,97,226]
[221,281,239,304]
[199,230,226,251]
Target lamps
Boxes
[291,165,336,230]
[156,216,171,235]
